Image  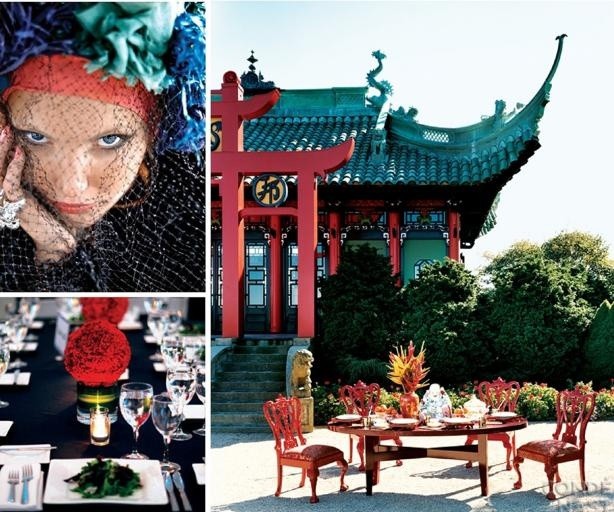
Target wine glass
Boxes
[0,325,10,407]
[119,311,205,460]
[4,319,28,353]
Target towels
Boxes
[1,457,44,512]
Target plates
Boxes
[390,418,418,423]
[43,459,169,505]
[336,415,362,420]
[492,412,517,417]
[445,418,471,424]
[0,444,51,465]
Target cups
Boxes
[90,407,110,446]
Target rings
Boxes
[0,184,26,229]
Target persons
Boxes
[0,3,206,293]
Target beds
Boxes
[1,316,204,512]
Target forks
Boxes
[22,464,33,504]
[7,470,19,502]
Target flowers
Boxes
[78,297,129,323]
[63,321,131,385]
[386,339,431,390]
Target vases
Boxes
[75,378,119,424]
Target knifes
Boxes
[172,472,193,511]
[13,368,21,386]
[163,472,180,512]
